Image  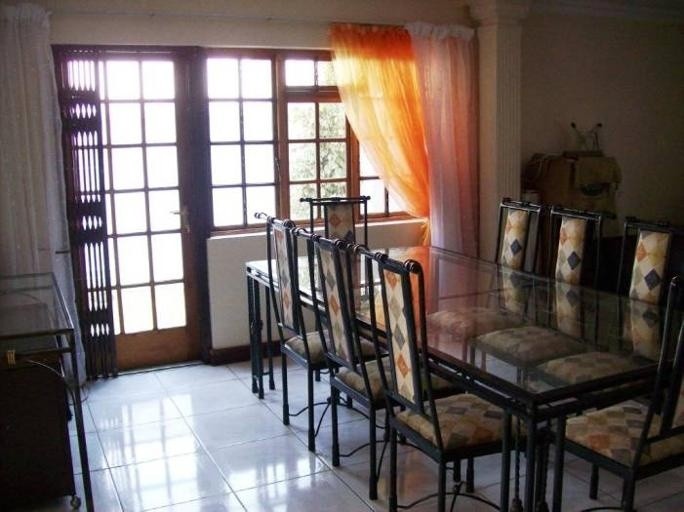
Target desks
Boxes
[244,244,681,510]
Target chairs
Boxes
[547,200,616,286]
[619,212,682,306]
[297,195,373,384]
[372,250,530,509]
[491,197,545,276]
[263,215,409,454]
[295,226,463,501]
[547,274,682,509]
